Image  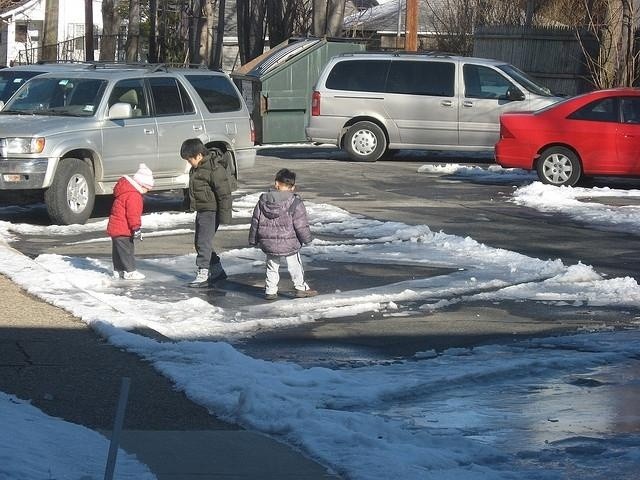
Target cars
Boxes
[494,86,639,187]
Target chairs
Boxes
[118,89,142,117]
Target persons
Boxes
[180,138,232,288]
[105,162,155,280]
[249,168,318,300]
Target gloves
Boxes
[132,230,144,241]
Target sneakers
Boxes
[208,262,227,284]
[265,291,280,300]
[113,269,145,279]
[295,289,318,298]
[189,267,212,288]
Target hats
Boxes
[133,163,154,190]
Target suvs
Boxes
[306,53,567,163]
